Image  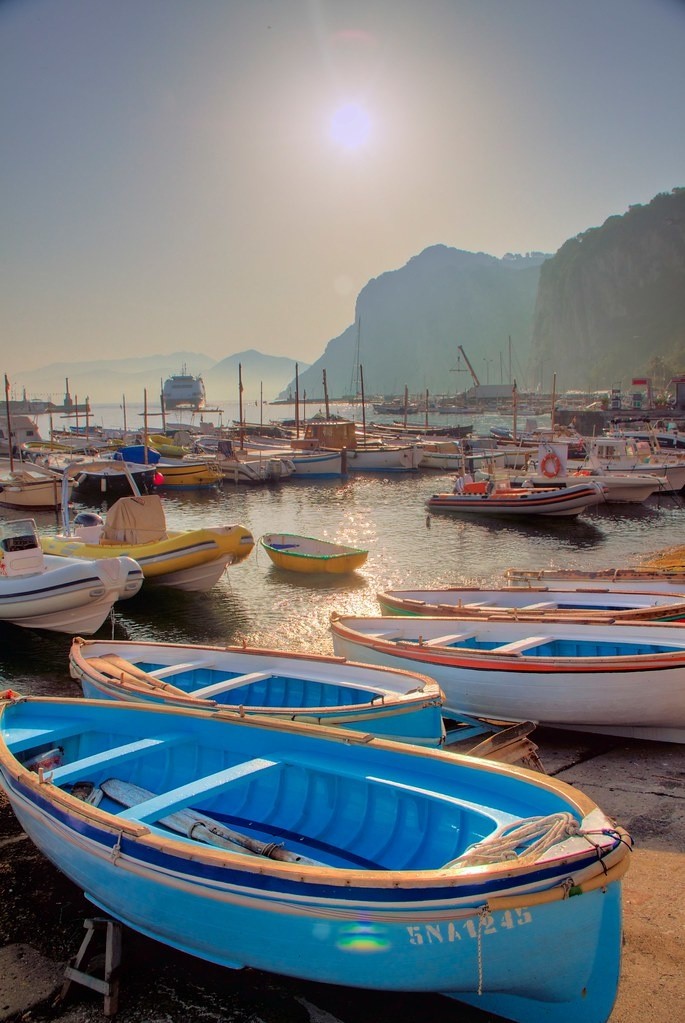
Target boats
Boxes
[424,436,608,519]
[375,586,685,622]
[38,458,256,593]
[162,362,207,411]
[0,517,147,638]
[0,689,634,1023]
[504,567,685,595]
[70,636,447,752]
[0,316,685,512]
[326,609,685,746]
[261,532,369,573]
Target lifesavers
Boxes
[540,453,561,479]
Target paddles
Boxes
[98,776,341,869]
[83,653,199,700]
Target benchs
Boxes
[520,601,559,609]
[44,731,195,786]
[491,634,557,652]
[114,750,303,825]
[190,671,273,698]
[463,601,495,608]
[139,661,214,683]
[422,631,479,646]
